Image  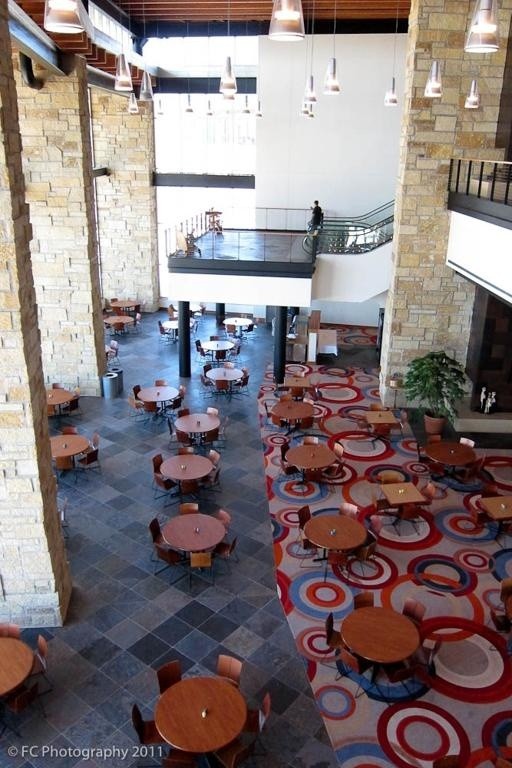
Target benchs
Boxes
[176,235,197,257]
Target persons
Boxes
[310,200,321,226]
